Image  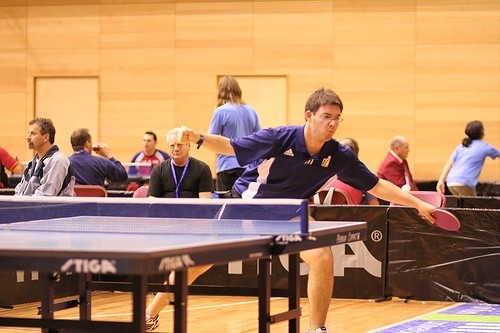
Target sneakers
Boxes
[145,312,160,330]
[306,326,325,333]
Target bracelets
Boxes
[197,134,204,150]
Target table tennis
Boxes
[402,184,411,193]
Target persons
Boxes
[436,120,500,197]
[377,136,420,192]
[146,126,215,198]
[316,137,381,206]
[142,88,439,333]
[0,146,26,190]
[13,117,77,198]
[68,127,128,186]
[207,75,262,199]
[128,132,172,191]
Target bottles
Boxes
[92,143,107,151]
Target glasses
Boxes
[313,113,344,123]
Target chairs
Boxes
[70,184,108,197]
[391,191,447,211]
[312,187,350,204]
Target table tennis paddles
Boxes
[421,209,460,231]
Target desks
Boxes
[0,197,368,333]
